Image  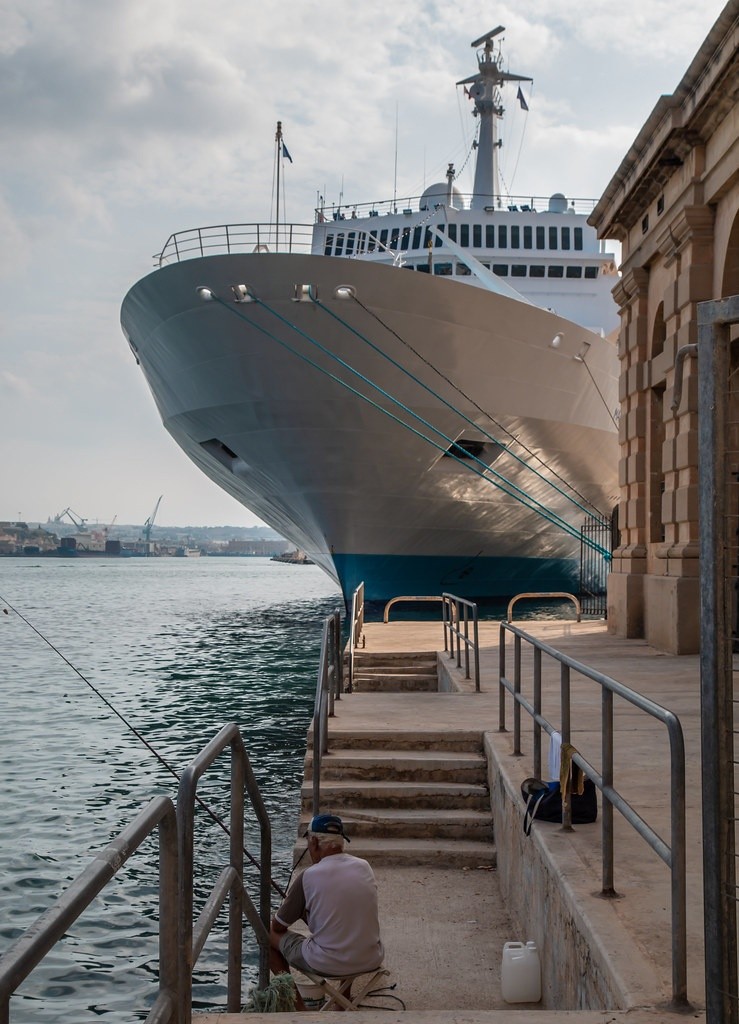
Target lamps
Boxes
[484,206,494,211]
[403,209,412,214]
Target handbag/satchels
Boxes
[520,775,597,837]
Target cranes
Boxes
[141,495,163,540]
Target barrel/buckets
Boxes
[500,941,542,1003]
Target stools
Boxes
[306,966,391,1010]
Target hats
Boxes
[302,815,351,843]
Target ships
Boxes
[117,23,631,615]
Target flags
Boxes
[283,143,292,163]
[517,86,529,111]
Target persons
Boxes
[269,813,386,1011]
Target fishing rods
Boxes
[0,595,292,901]
